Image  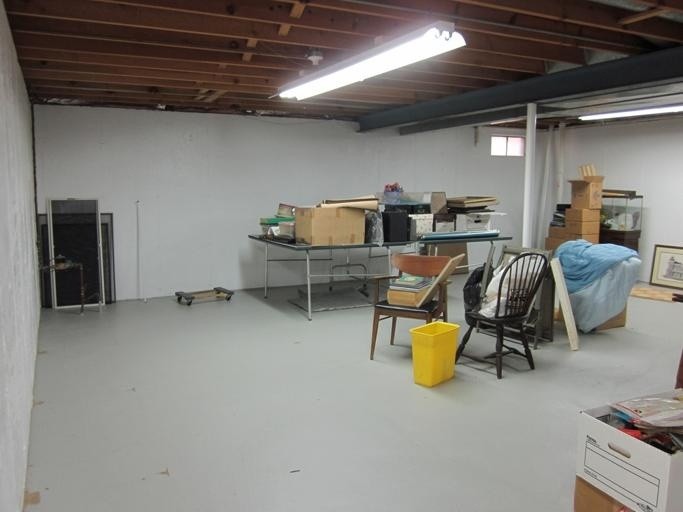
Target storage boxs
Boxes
[570,403,683,512]
[544,174,603,249]
[277,194,507,245]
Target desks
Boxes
[249,230,500,322]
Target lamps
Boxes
[267,18,468,102]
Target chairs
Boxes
[368,251,453,361]
[455,251,548,380]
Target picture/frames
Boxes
[647,244,683,290]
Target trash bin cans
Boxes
[408,321,460,388]
